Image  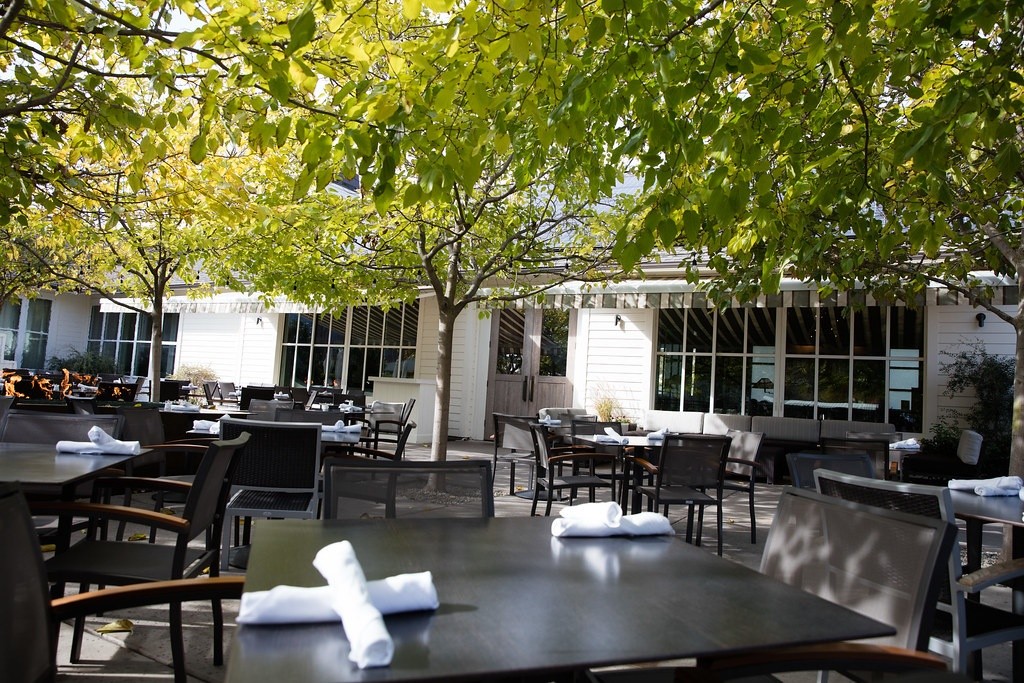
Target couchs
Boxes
[538,407,608,469]
[626,409,903,480]
[920,431,983,484]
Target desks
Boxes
[159,408,258,477]
[572,434,711,547]
[293,406,364,474]
[224,516,898,683]
[951,490,1024,683]
[185,422,361,572]
[0,441,152,683]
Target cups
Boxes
[322,403,329,412]
[621,423,629,436]
[164,400,172,412]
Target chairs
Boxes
[0,367,1024,683]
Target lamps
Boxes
[976,313,986,327]
[613,314,622,326]
[256,315,263,326]
